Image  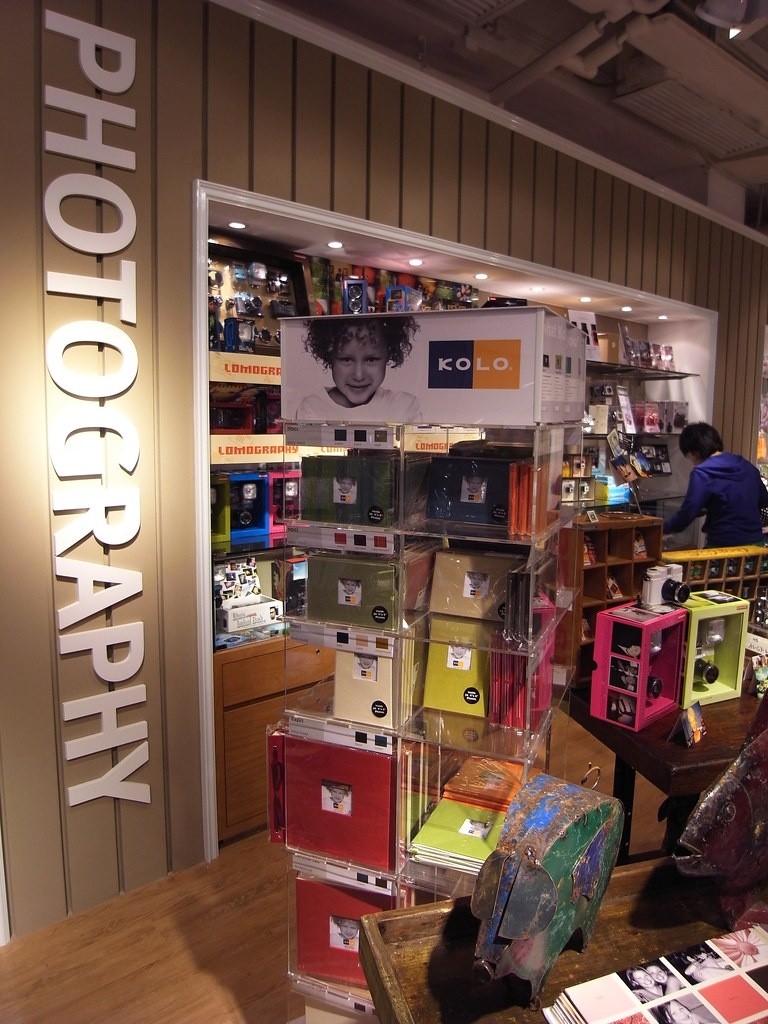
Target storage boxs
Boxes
[590,601,687,732]
[216,594,283,632]
[680,590,750,710]
[220,470,269,539]
[269,469,302,533]
[211,475,230,544]
[631,401,688,433]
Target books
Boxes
[266,440,542,1024]
[543,925,768,1024]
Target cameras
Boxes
[274,480,297,524]
[642,564,690,606]
[230,483,258,528]
[647,676,662,698]
[694,642,719,685]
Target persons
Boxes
[626,966,667,1003]
[663,422,768,547]
[686,707,704,745]
[337,578,362,604]
[354,653,377,682]
[321,778,351,815]
[464,819,490,839]
[295,315,424,422]
[462,571,490,598]
[447,640,470,669]
[461,476,485,503]
[222,558,280,624]
[333,475,356,503]
[682,954,734,982]
[658,999,719,1024]
[636,962,685,1000]
[329,916,359,951]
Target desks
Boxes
[545,657,768,867]
[359,855,768,1024]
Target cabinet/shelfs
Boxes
[561,360,700,509]
[273,305,592,1024]
[554,511,768,676]
[209,350,337,842]
